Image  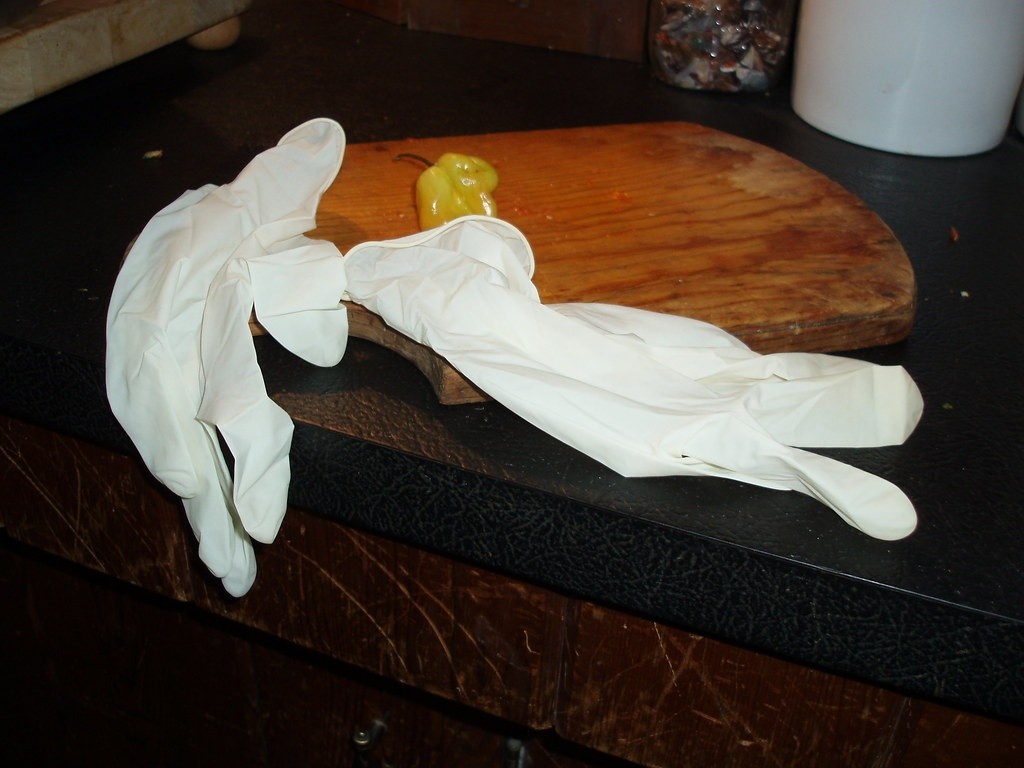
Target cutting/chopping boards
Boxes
[244,120,919,405]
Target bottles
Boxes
[644,0,793,105]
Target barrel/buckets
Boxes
[788,0,1024,158]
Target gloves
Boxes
[106,117,349,598]
[345,218,922,542]
